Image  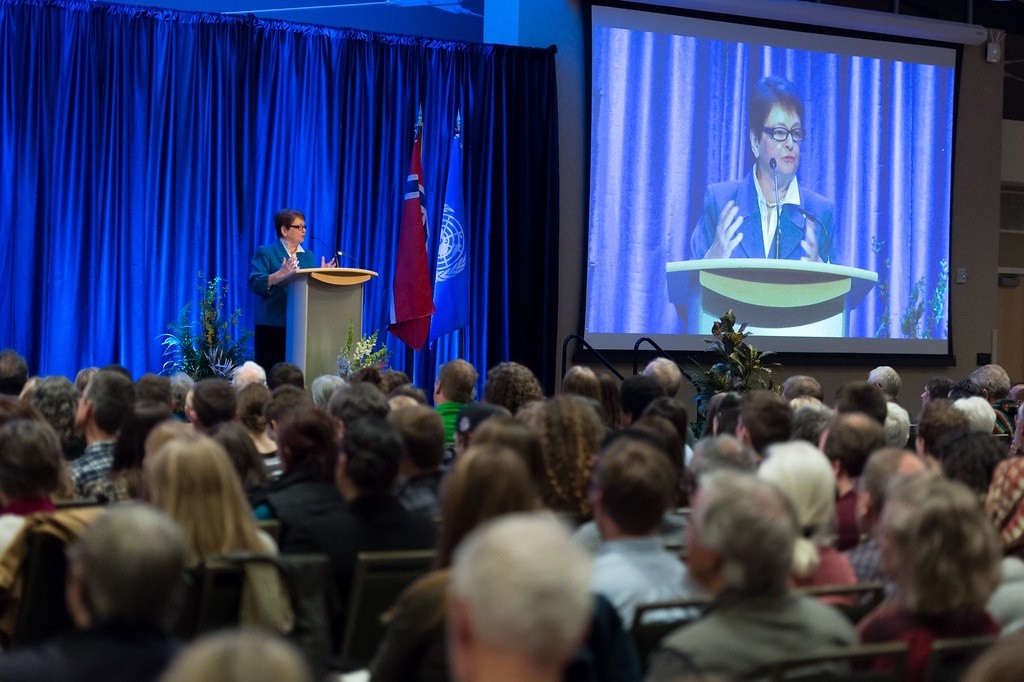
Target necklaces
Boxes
[766,198,785,208]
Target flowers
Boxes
[336,318,392,377]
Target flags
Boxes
[386,124,437,351]
[428,131,473,351]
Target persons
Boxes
[0,349,1024,682]
[247,210,337,367]
[690,75,841,260]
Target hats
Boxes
[454,401,491,433]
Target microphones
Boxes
[309,235,339,268]
[770,158,782,259]
[797,207,832,264]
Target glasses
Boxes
[288,224,309,232]
[759,124,807,143]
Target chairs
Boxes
[54,486,995,682]
